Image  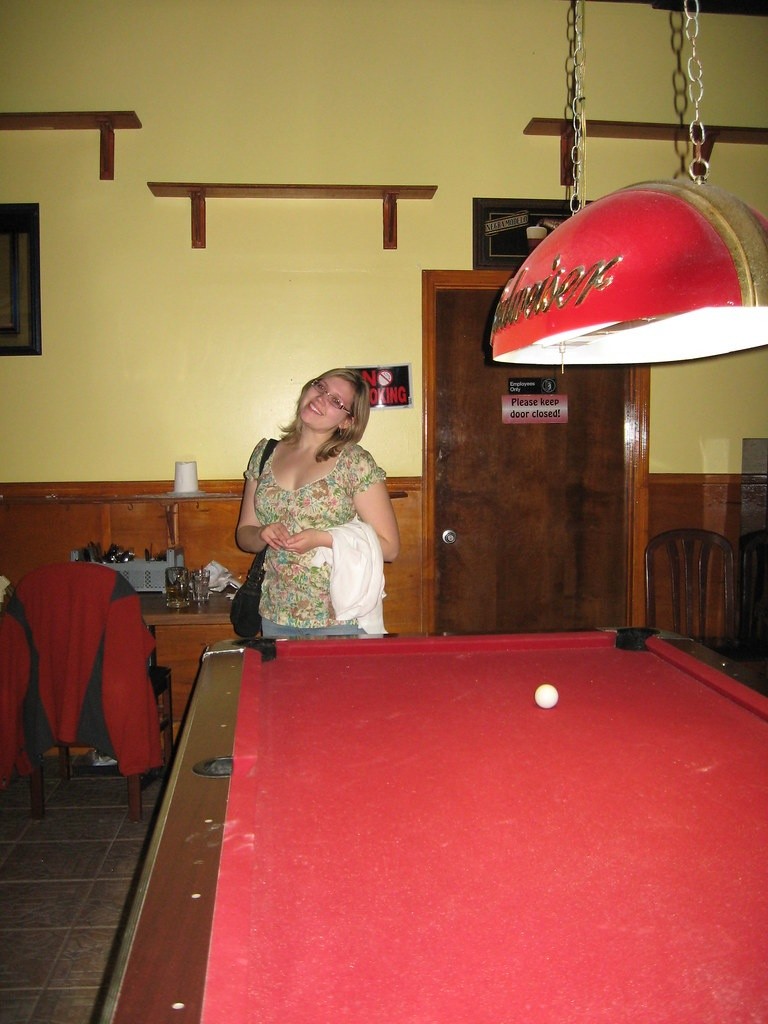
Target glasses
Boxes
[311,378,354,418]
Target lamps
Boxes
[489,0,768,368]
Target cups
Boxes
[165,566,190,608]
[190,569,209,601]
[175,461,197,491]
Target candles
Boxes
[174,461,199,493]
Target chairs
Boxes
[735,530,768,661]
[644,527,747,661]
[0,559,174,820]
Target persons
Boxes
[236,368,400,637]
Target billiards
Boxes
[534,682,561,710]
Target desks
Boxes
[43,585,262,756]
[96,625,768,1024]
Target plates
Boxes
[166,491,207,498]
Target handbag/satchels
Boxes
[230,542,269,638]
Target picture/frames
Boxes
[473,197,593,271]
[0,203,42,357]
